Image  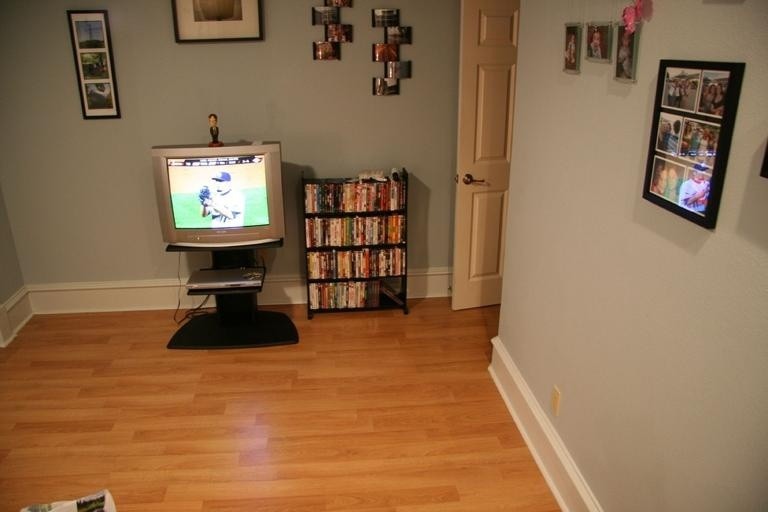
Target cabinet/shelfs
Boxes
[164,238,297,348]
[305,170,411,324]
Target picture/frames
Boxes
[612,20,639,83]
[170,0,264,43]
[639,58,751,232]
[562,24,579,74]
[584,21,613,63]
[62,11,123,119]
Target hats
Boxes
[212,172,231,181]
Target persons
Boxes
[589,31,602,57]
[198,170,246,227]
[208,113,220,143]
[649,66,732,218]
[618,32,630,76]
[566,33,576,65]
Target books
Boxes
[303,182,407,310]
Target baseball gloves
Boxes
[200,189,209,206]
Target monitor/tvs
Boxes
[151,141,285,246]
